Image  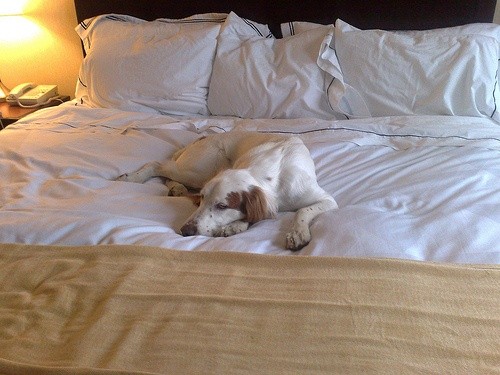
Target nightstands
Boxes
[0,94,73,130]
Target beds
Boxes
[0,0,499,375]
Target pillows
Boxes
[331,17,500,128]
[207,9,371,122]
[73,12,230,115]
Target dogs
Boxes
[110,129,338,252]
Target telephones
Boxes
[6,82,58,107]
